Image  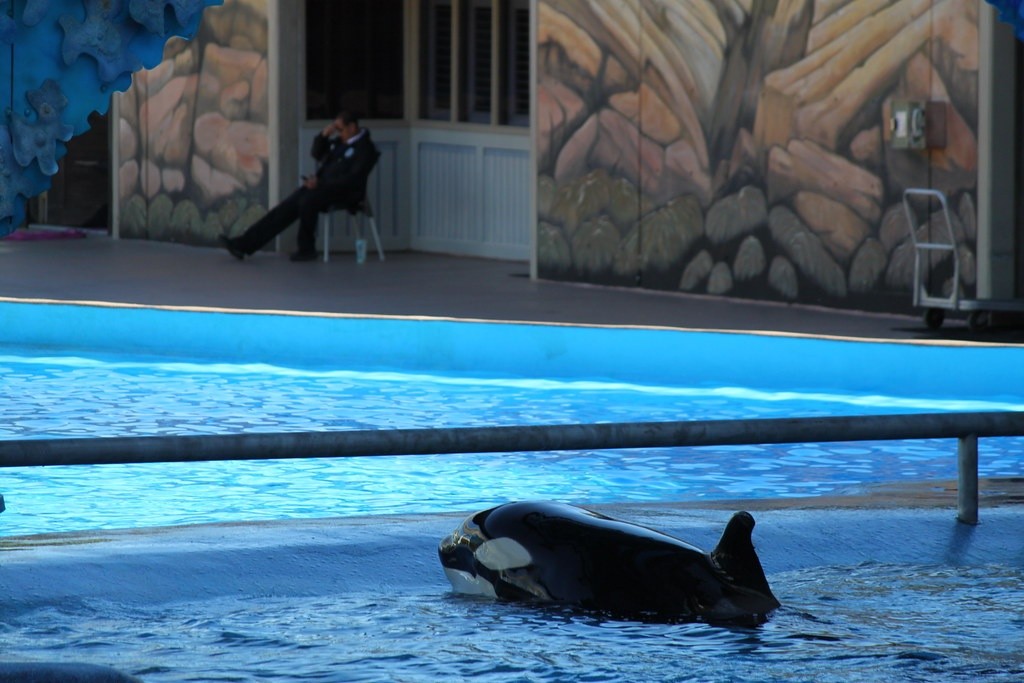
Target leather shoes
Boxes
[290,250,318,261]
[217,233,247,261]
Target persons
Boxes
[218,109,377,262]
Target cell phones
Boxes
[301,175,308,180]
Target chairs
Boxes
[314,150,386,263]
